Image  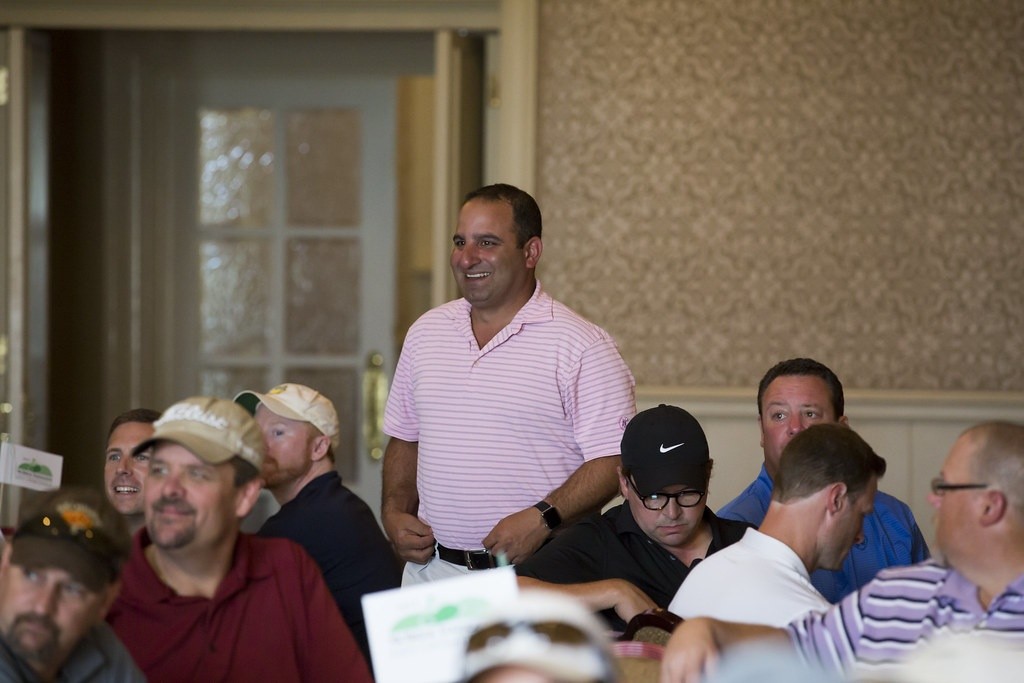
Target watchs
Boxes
[533,501,562,530]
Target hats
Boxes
[446,587,615,683]
[232,383,342,455]
[131,397,268,474]
[620,404,709,497]
[8,489,132,592]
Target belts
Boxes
[431,539,498,570]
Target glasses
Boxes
[931,479,987,497]
[626,474,705,511]
[15,509,116,560]
[465,620,592,648]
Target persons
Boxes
[460,589,615,683]
[716,357,931,605]
[381,183,638,587]
[105,408,162,537]
[514,404,759,632]
[666,423,886,628]
[660,421,1024,683]
[0,486,147,683]
[232,383,401,683]
[105,395,374,683]
